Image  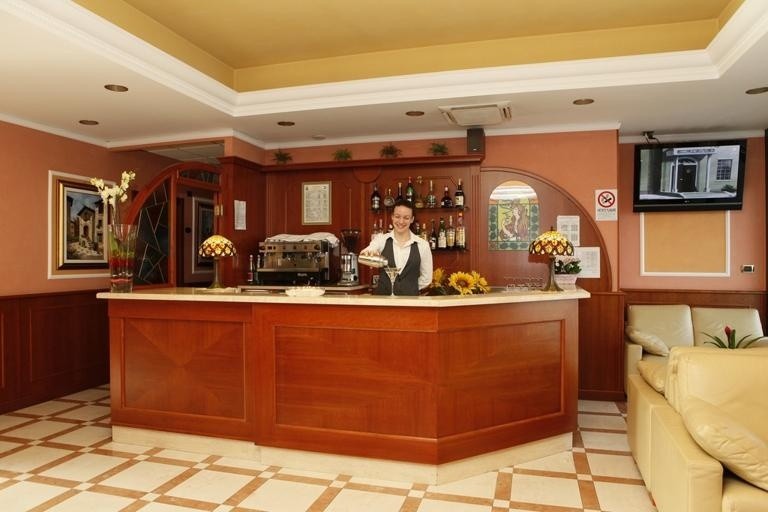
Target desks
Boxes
[241,281,373,295]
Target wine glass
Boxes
[384,267,401,297]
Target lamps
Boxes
[197,232,237,289]
[528,226,576,293]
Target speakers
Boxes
[467,127,486,155]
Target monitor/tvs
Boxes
[632,138,747,212]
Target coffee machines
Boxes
[258,240,331,284]
[338,228,361,286]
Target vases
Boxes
[105,220,139,292]
[553,272,579,293]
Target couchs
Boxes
[619,340,767,460]
[626,348,767,512]
[622,297,768,399]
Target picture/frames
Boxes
[299,178,334,227]
[190,195,213,277]
[45,166,110,279]
[640,211,733,280]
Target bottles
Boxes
[247,253,261,285]
[371,176,464,208]
[357,254,389,268]
[371,212,466,249]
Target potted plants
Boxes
[272,140,449,166]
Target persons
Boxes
[357,199,433,295]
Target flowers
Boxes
[89,166,136,258]
[699,322,764,353]
[429,263,490,295]
[553,256,583,276]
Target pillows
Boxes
[678,394,768,489]
[626,325,671,359]
[636,355,673,393]
